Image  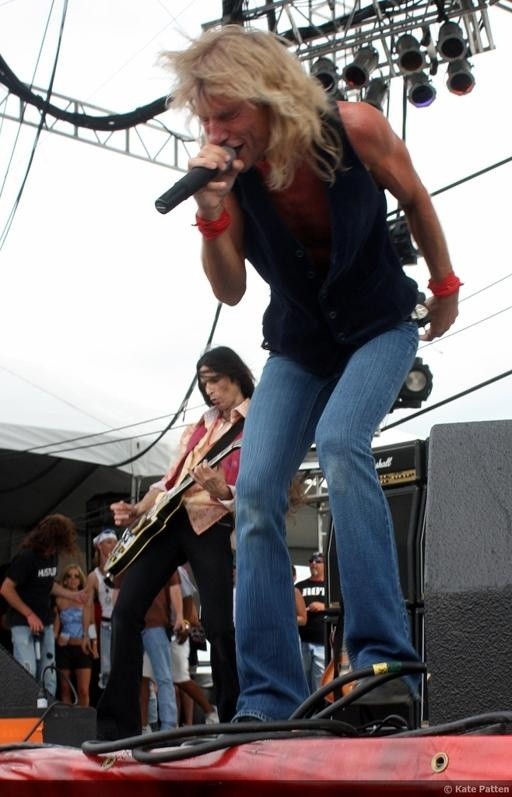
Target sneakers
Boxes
[141,724,153,735]
[204,705,220,724]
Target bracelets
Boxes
[189,209,232,240]
[425,270,464,302]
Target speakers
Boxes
[0,646,56,719]
[322,440,426,605]
[320,614,422,725]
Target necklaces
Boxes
[216,415,231,431]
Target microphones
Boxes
[154,145,236,215]
[32,631,42,660]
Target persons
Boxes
[80,528,118,692]
[55,563,92,708]
[149,22,465,724]
[140,560,221,735]
[289,563,308,627]
[91,343,258,741]
[293,550,333,694]
[0,512,91,701]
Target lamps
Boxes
[309,13,477,113]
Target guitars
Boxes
[104,439,241,577]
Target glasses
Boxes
[64,573,81,579]
[96,529,116,543]
[309,559,323,563]
